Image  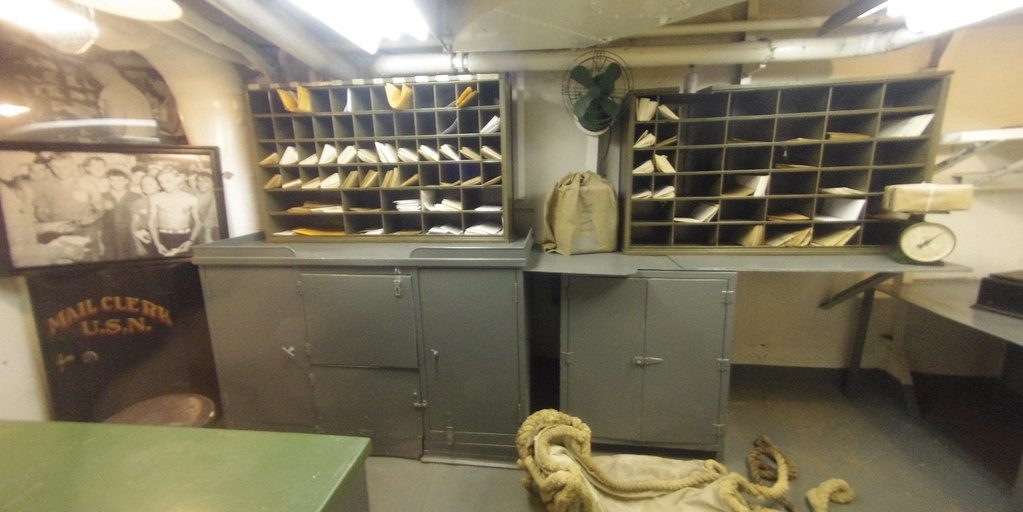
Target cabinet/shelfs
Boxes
[245,78,517,244]
[619,70,955,256]
[559,270,737,463]
[191,226,534,470]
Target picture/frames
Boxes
[0,141,229,276]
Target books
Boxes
[632,97,679,199]
[261,139,500,213]
[673,175,863,246]
[826,132,870,140]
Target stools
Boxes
[102,395,217,428]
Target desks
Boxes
[874,279,1023,347]
[0,421,373,512]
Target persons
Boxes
[0,153,219,268]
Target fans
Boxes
[561,50,633,135]
